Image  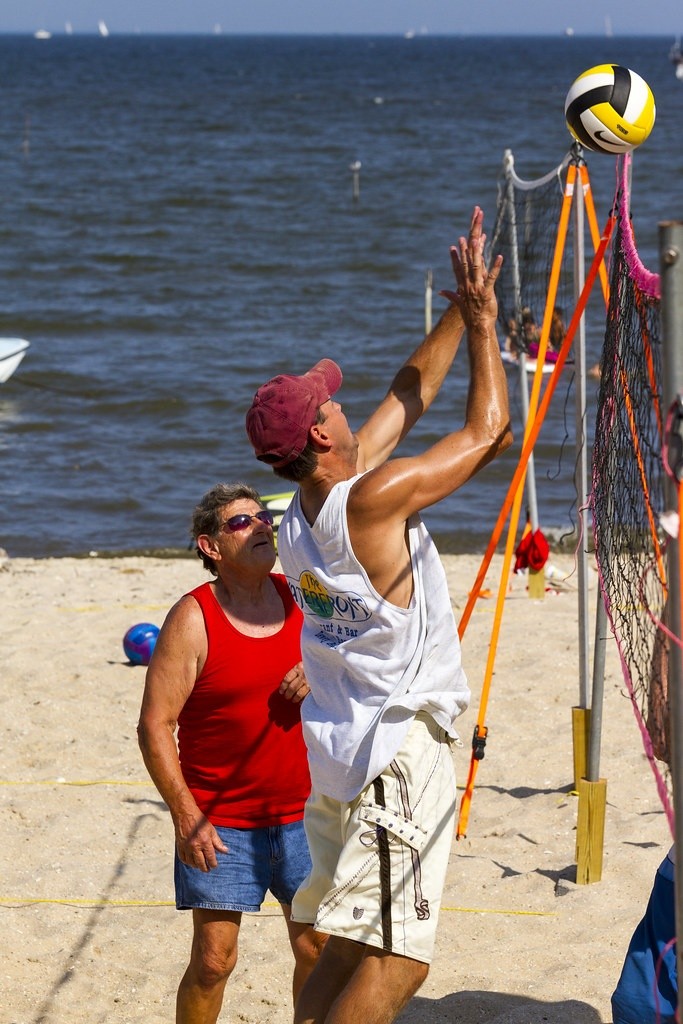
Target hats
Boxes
[246,358,343,467]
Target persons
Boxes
[506,305,566,360]
[247,206,516,1024]
[138,484,328,1024]
[611,590,683,1024]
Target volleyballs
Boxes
[562,61,659,156]
[121,620,162,666]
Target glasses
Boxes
[213,510,274,530]
